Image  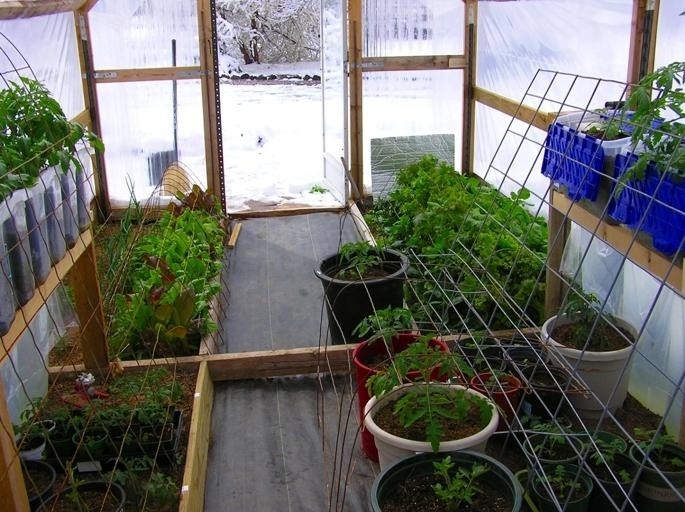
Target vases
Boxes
[503,347,550,378]
[21,460,58,512]
[520,364,570,421]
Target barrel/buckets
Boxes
[361,381,502,473]
[351,332,452,463]
[541,309,640,421]
[313,247,412,346]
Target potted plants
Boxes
[541,288,640,421]
[70,410,108,460]
[532,463,594,512]
[48,409,73,460]
[11,421,47,462]
[363,336,501,474]
[19,397,56,436]
[572,429,628,463]
[138,400,176,464]
[630,427,685,512]
[471,373,522,429]
[580,452,637,510]
[307,185,409,345]
[36,466,126,512]
[523,420,584,470]
[351,306,447,461]
[468,355,507,373]
[458,339,500,358]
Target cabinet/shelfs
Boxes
[0,223,109,512]
[545,182,685,512]
